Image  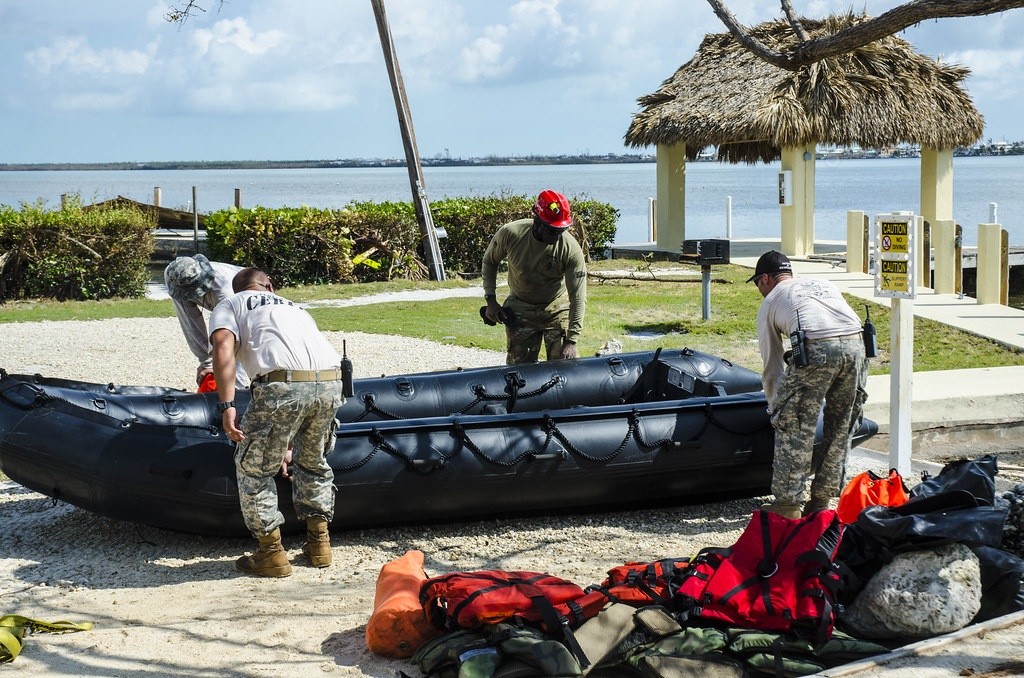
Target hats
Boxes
[164,254,217,302]
[745,250,792,283]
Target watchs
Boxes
[217,401,236,414]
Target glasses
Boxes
[754,272,767,287]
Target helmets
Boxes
[531,190,573,227]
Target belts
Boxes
[804,331,863,343]
[255,368,342,381]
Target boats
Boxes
[0,348,878,539]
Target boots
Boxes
[235,525,292,577]
[302,516,332,567]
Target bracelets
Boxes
[485,294,496,300]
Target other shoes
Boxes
[761,502,801,519]
[801,500,829,517]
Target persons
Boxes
[482,190,587,366]
[209,268,348,578]
[164,254,251,389]
[745,248,868,519]
